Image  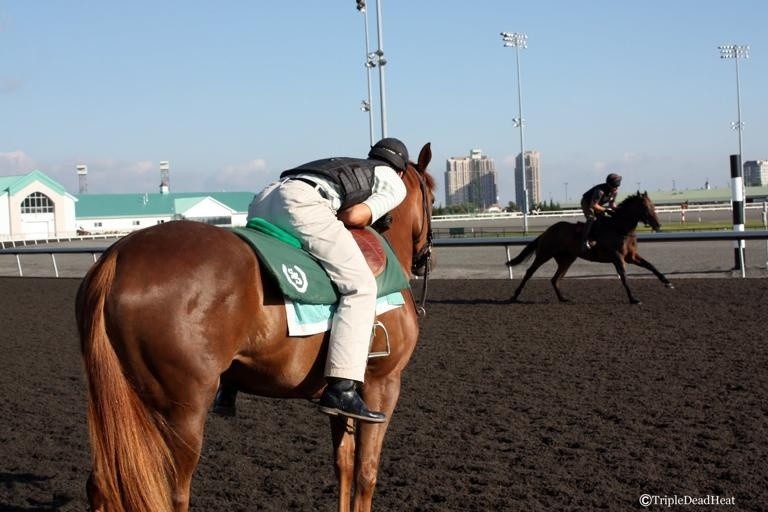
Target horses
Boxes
[75,141,433,512]
[504,189,674,306]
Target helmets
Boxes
[605,174,621,186]
[368,137,408,172]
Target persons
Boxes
[243,134,412,423]
[579,173,623,254]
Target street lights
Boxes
[353,0,386,150]
[498,28,530,236]
[714,42,751,225]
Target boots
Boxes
[576,221,596,246]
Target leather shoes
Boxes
[316,378,386,423]
[212,386,236,417]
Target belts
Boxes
[289,177,327,199]
